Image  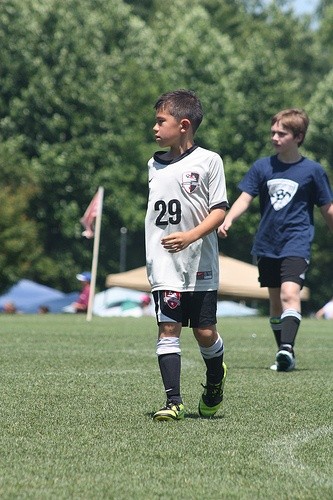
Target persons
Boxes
[2,301,23,315]
[145,88,231,420]
[61,272,91,314]
[315,299,333,318]
[217,108,333,372]
[38,305,50,314]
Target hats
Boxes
[76,271,90,282]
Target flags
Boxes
[79,186,104,238]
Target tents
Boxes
[106,253,310,308]
[1,278,81,315]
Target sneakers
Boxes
[153,401,186,421]
[270,359,297,370]
[276,345,294,369]
[198,361,228,417]
